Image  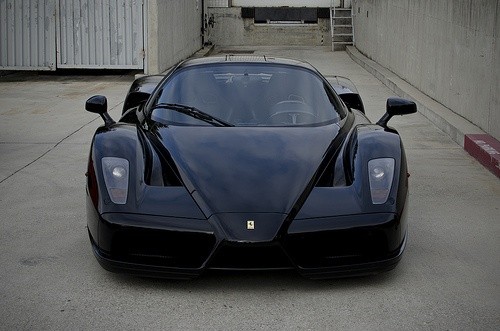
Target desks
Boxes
[254,7,317,24]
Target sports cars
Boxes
[84,54,417,280]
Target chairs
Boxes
[175,71,226,126]
[264,73,313,126]
